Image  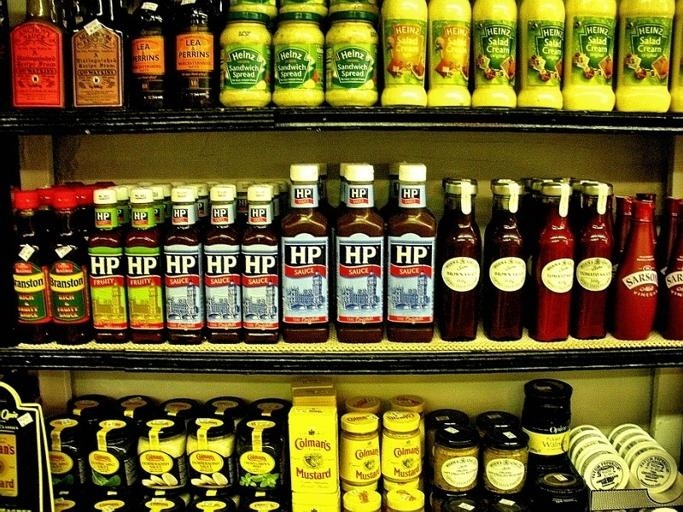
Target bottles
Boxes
[8,159,682,345]
[0,1,683,112]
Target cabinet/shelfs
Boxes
[0,0,682,512]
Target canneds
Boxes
[42,395,292,512]
[339,378,589,512]
[219,0,379,107]
